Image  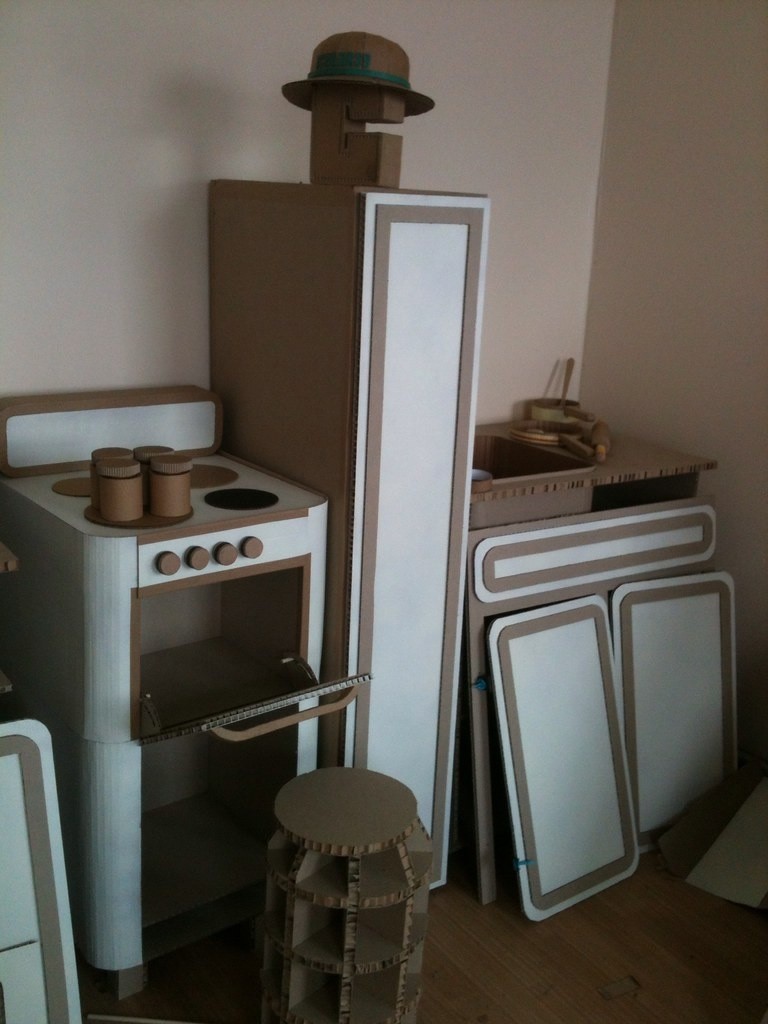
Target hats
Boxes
[281,30,434,118]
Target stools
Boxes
[259,769,436,1024]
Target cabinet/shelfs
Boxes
[1,719,84,1024]
[207,172,492,890]
[465,490,743,921]
